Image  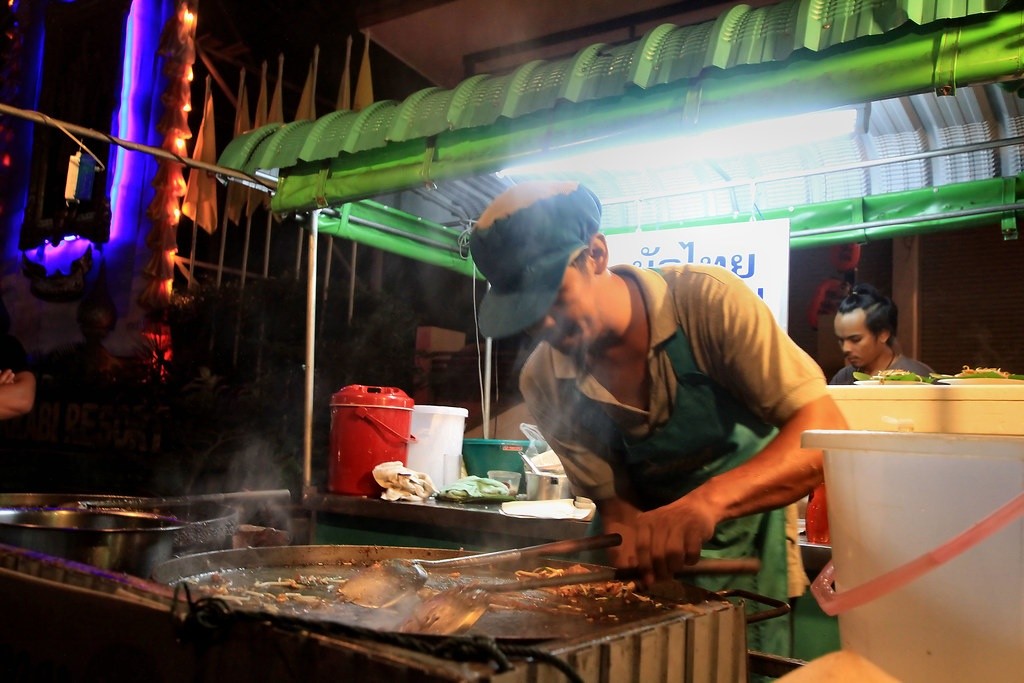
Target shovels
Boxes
[339,532,624,610]
[50,487,292,513]
[389,558,762,637]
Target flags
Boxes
[224,54,284,225]
[181,74,218,234]
[335,36,353,111]
[295,45,320,123]
[353,30,375,112]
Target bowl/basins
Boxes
[461,438,541,495]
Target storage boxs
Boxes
[460,437,551,491]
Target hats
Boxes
[470,181,602,338]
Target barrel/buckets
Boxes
[327,384,416,499]
[405,404,469,490]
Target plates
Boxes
[853,381,931,385]
[938,378,1024,385]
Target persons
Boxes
[469,182,854,683]
[0,367,38,420]
[828,285,940,385]
